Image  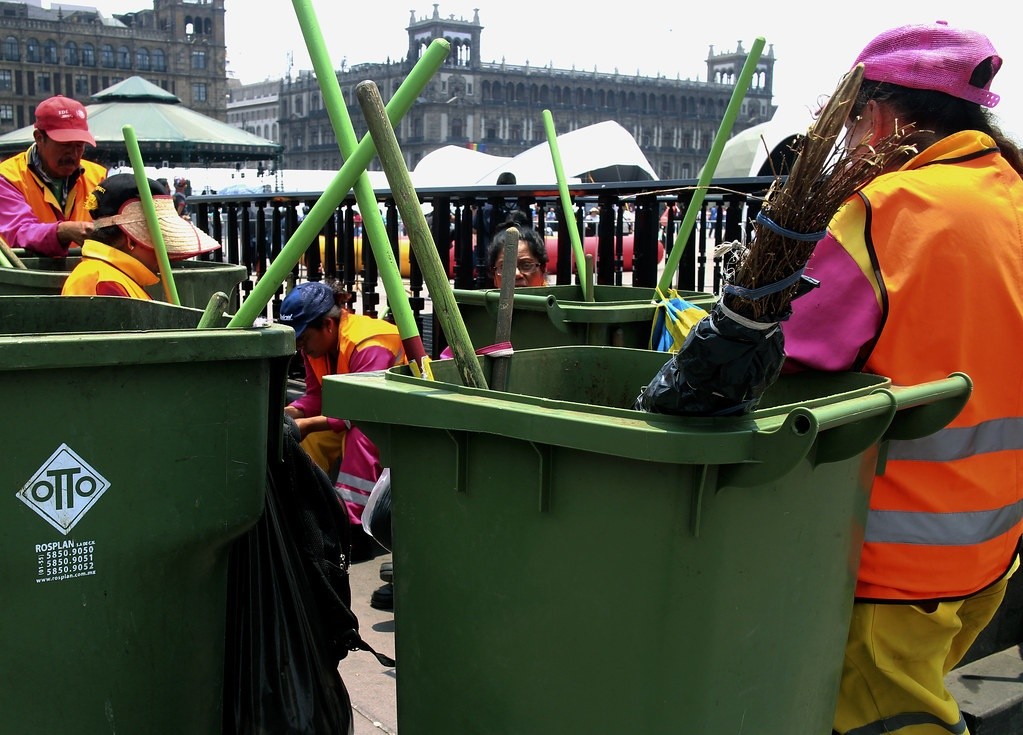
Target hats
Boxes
[89,196,221,261]
[279,282,335,338]
[88,174,169,217]
[815,20,1003,117]
[34,94,97,148]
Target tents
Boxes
[695,120,772,178]
[109,168,415,197]
[413,120,659,188]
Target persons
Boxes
[157,179,170,194]
[172,176,190,223]
[0,96,108,260]
[545,200,727,249]
[782,20,1023,735]
[277,212,551,614]
[61,173,222,301]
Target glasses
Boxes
[494,262,541,275]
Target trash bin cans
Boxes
[0,241,972,735]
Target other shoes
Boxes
[371,583,393,609]
[380,561,393,582]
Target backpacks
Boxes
[648,288,708,353]
